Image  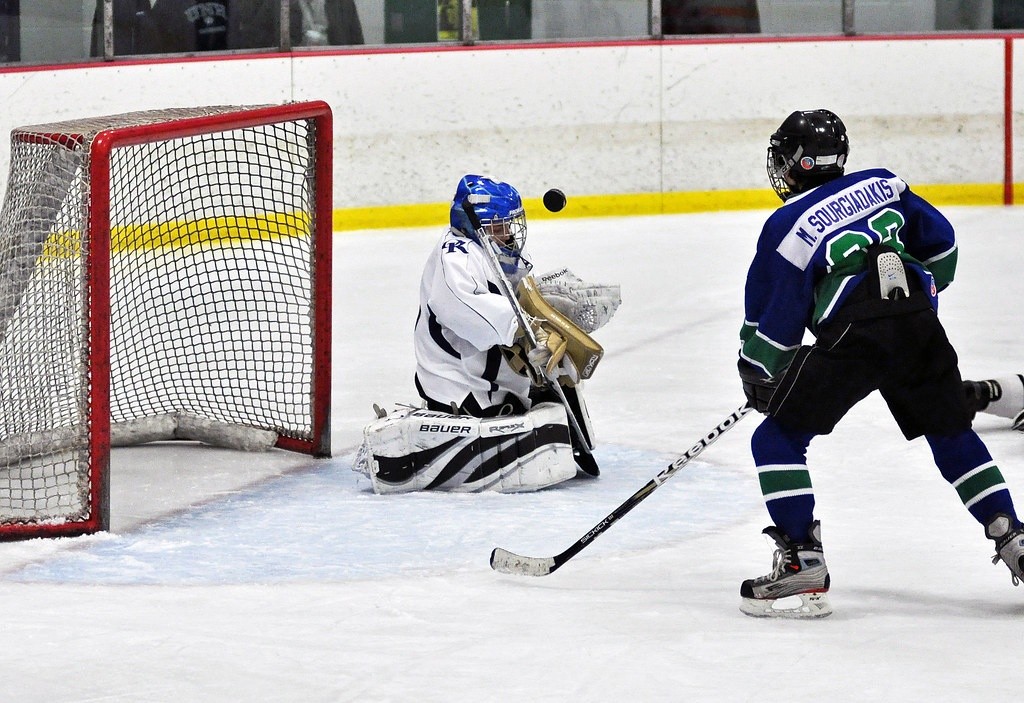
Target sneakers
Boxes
[984,513,1024,587]
[962,374,1024,420]
[1011,409,1024,431]
[736,519,833,619]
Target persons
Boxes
[89,0,365,59]
[350,174,600,493]
[740,109,1024,620]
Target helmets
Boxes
[766,108,850,203]
[449,174,527,274]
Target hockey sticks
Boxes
[491,398,754,578]
[465,194,599,477]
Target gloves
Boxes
[527,326,551,367]
[742,381,776,414]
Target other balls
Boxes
[543,187,567,213]
